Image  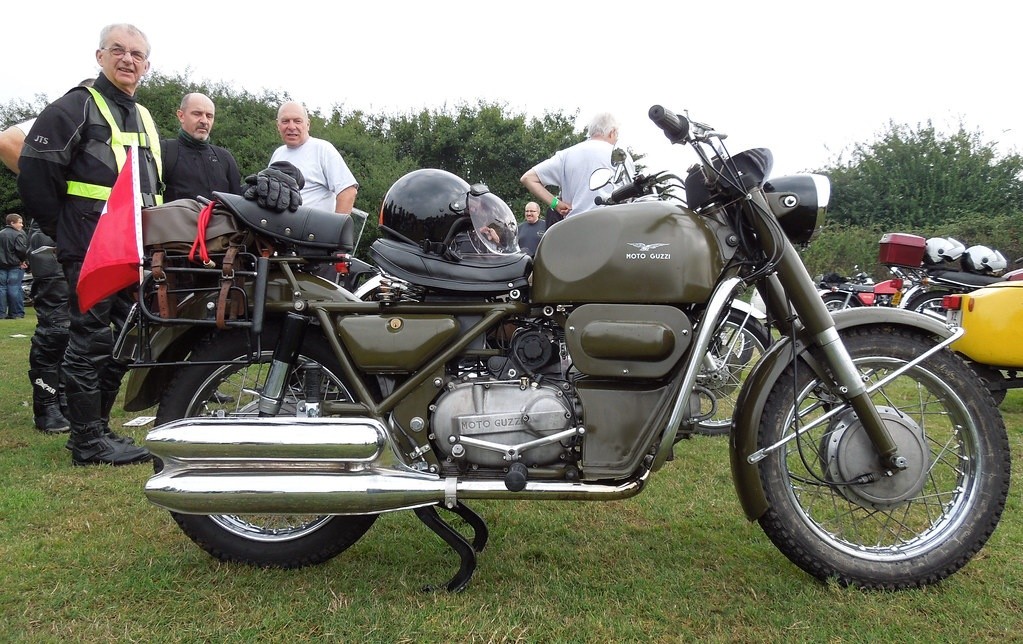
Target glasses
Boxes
[102,46,148,62]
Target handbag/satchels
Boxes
[142,196,250,254]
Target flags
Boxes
[77,146,141,314]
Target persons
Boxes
[268,102,360,283]
[518,202,546,253]
[479,223,503,248]
[0,214,28,319]
[0,78,96,434]
[159,93,242,204]
[519,114,635,219]
[545,191,564,232]
[16,23,166,468]
[23,228,56,306]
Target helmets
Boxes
[922,236,966,271]
[960,245,1007,275]
[379,169,521,262]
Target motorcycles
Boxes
[124,104,1013,595]
[818,231,1022,407]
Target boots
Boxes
[65,389,151,466]
[66,389,134,449]
[28,369,71,432]
[58,372,69,420]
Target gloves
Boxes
[243,162,306,214]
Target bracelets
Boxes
[549,197,559,211]
